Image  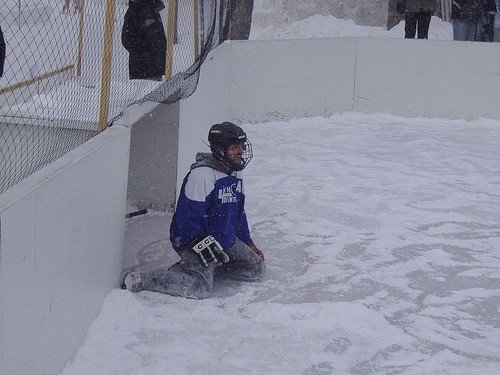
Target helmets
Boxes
[208,122,253,172]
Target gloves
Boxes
[249,243,264,262]
[188,232,229,268]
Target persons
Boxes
[397,0,437,39]
[450,0,498,42]
[122,121,265,300]
[122,0,166,83]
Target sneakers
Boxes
[123,267,145,292]
